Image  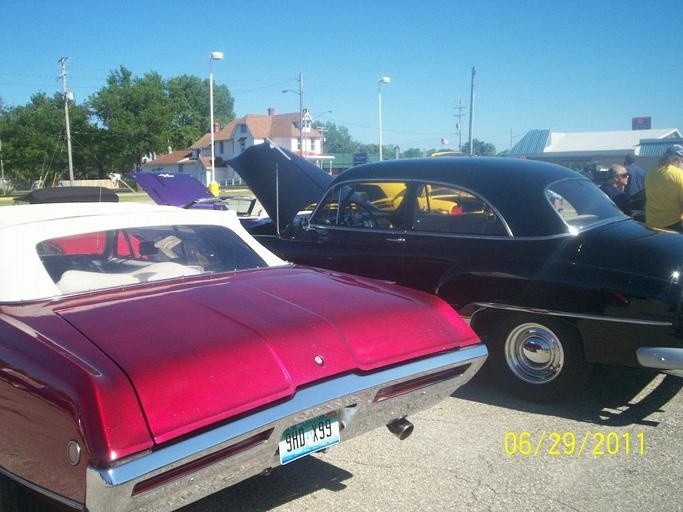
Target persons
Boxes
[600,164,632,217]
[644,144,683,234]
[624,154,646,208]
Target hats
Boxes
[666,144,683,156]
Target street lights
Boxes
[376,76,391,160]
[206,51,224,194]
[281,72,303,157]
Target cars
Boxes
[220,139,682,408]
[129,172,270,230]
[0,199,489,512]
[303,149,488,217]
[11,185,159,269]
